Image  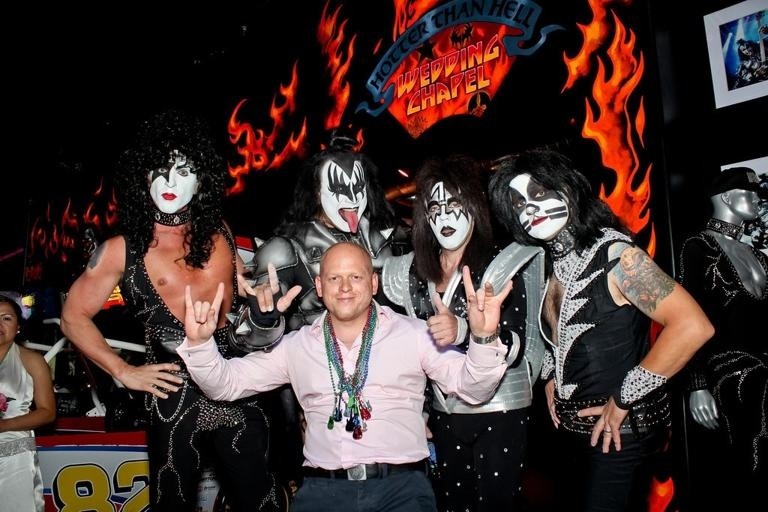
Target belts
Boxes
[554,393,672,435]
[303,463,425,480]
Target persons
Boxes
[174,242,512,512]
[674,168,768,512]
[59,113,279,512]
[488,151,717,512]
[731,26,768,87]
[373,159,545,512]
[0,296,57,512]
[224,150,412,438]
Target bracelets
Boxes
[470,329,498,344]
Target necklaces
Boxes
[323,303,377,439]
[153,210,191,226]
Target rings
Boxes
[603,430,612,434]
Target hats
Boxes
[710,166,764,198]
